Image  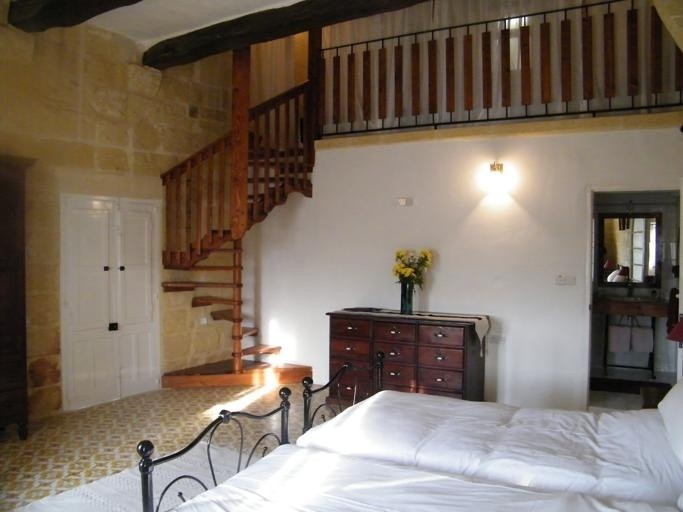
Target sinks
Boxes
[607,295,656,302]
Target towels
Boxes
[631,326,654,352]
[608,325,632,355]
[665,288,681,331]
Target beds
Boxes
[138,349,683,512]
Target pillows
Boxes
[657,380,683,465]
[607,269,620,282]
[612,275,626,283]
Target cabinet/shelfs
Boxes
[327,307,485,405]
[593,294,668,379]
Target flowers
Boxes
[390,249,432,290]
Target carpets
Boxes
[15,438,257,512]
[643,382,672,409]
[590,379,641,395]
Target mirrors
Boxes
[595,212,662,290]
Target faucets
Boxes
[627,280,634,296]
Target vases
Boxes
[399,279,415,314]
